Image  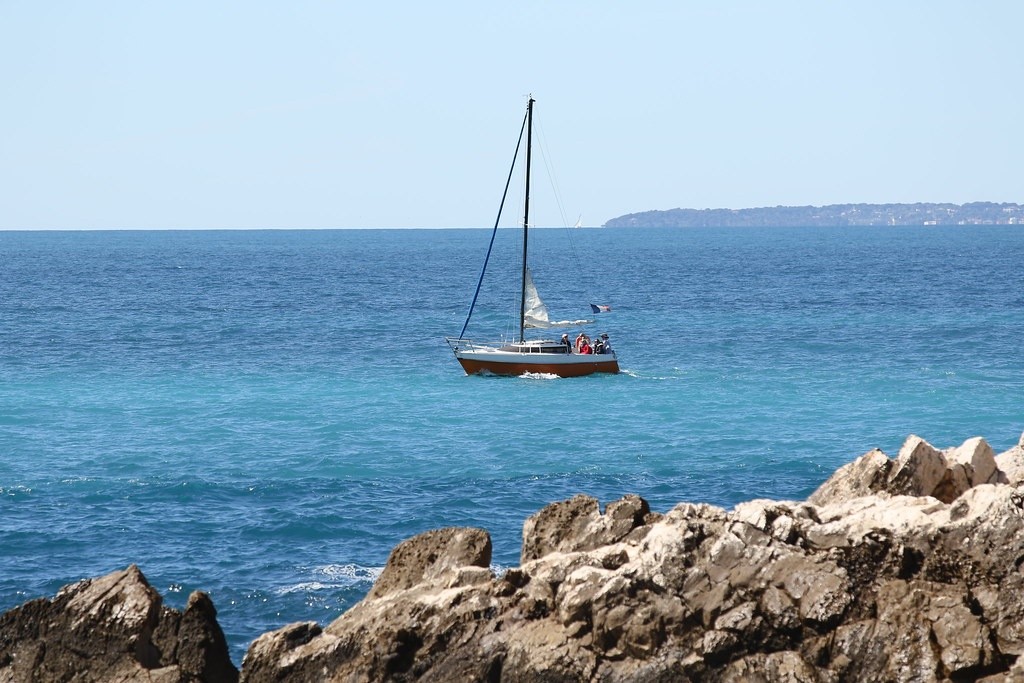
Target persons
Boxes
[594,333,611,354]
[560,334,571,352]
[575,333,593,354]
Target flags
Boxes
[591,303,611,314]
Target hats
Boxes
[601,334,609,338]
[562,334,568,338]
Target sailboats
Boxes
[444,94,619,379]
